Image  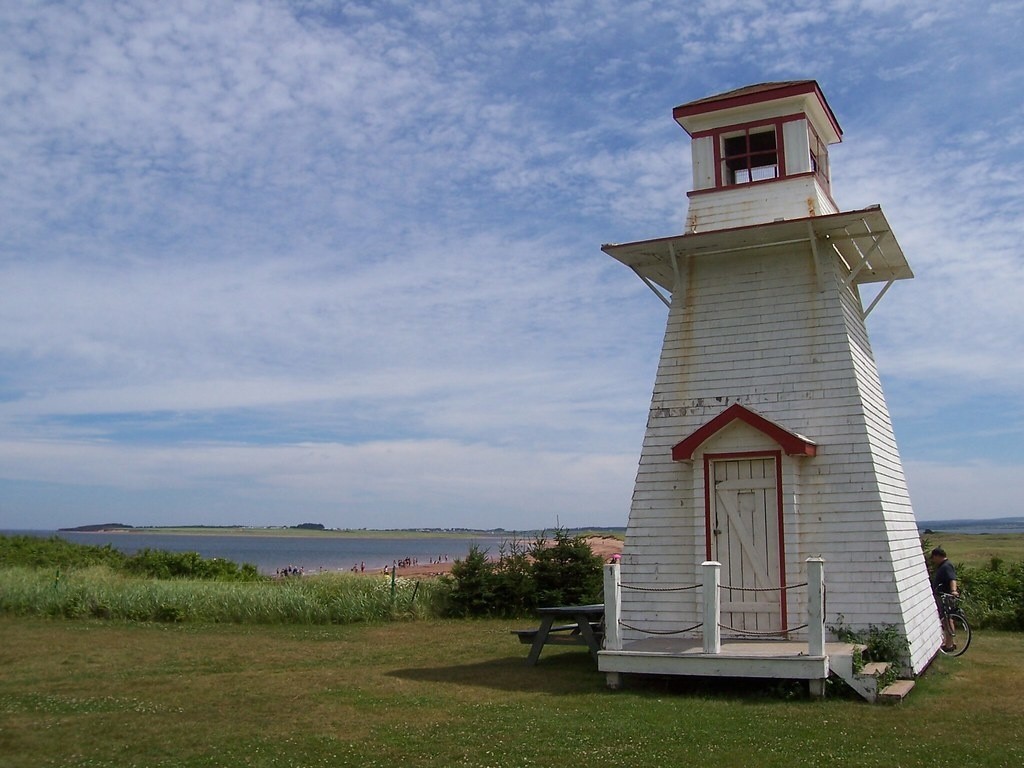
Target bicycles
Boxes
[933,583,972,658]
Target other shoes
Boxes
[940,644,957,652]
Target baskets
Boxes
[941,594,964,613]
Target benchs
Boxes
[510,621,598,636]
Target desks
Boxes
[525,604,604,667]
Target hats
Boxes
[929,549,946,559]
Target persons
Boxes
[428,553,448,565]
[928,548,959,652]
[384,565,390,576]
[325,570,327,571]
[351,561,365,574]
[320,566,323,572]
[276,563,304,577]
[393,556,418,568]
